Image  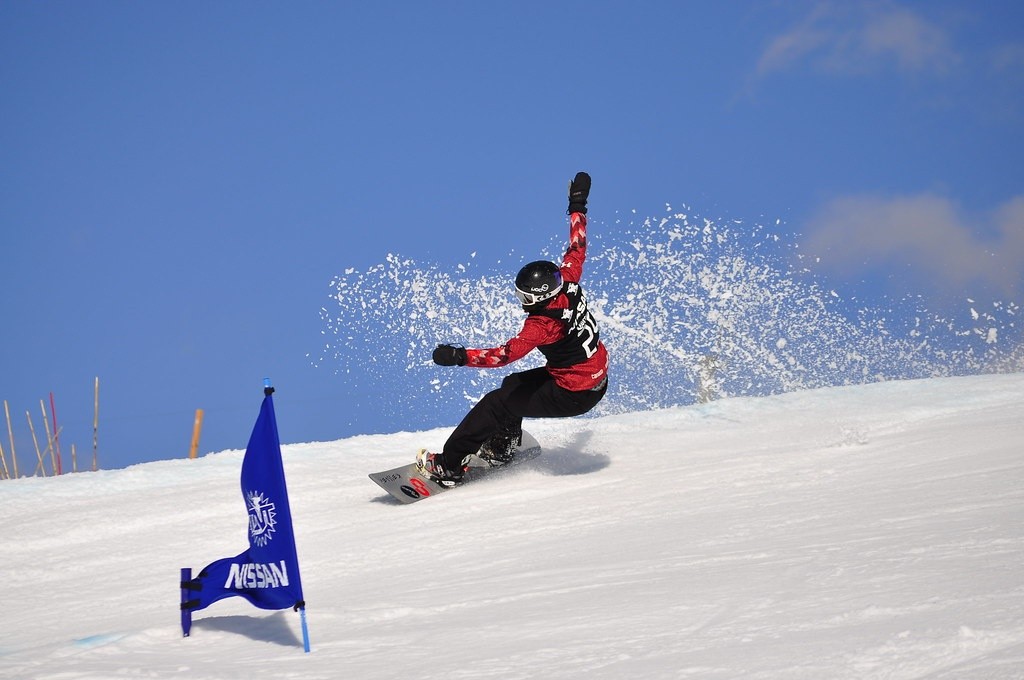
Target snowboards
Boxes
[366,427,542,504]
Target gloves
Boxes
[432,343,468,367]
[566,171,592,215]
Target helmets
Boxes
[515,260,563,313]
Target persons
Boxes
[415,172,611,489]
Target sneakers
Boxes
[415,447,465,489]
[475,443,515,468]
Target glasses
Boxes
[515,288,533,304]
